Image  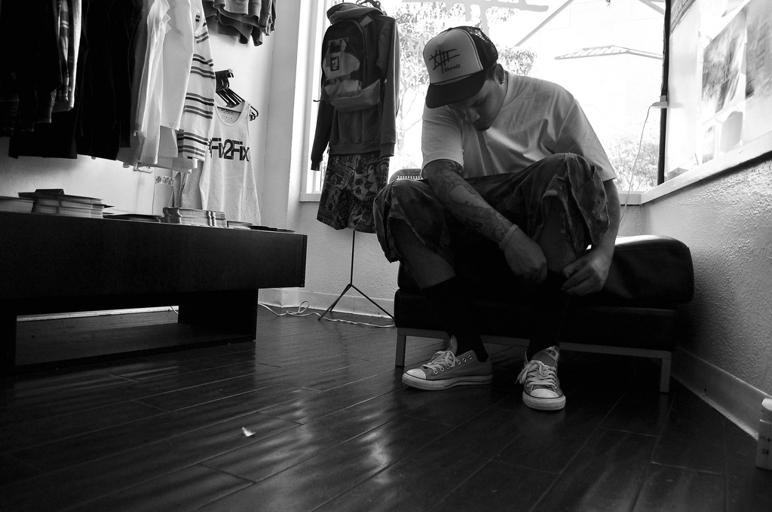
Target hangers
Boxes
[214,68,259,122]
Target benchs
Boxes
[0,211,307,377]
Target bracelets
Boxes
[497,224,518,250]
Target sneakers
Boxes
[522,347,566,411]
[401,350,492,391]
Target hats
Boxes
[422,26,498,109]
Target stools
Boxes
[386,232,693,393]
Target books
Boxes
[0,192,252,230]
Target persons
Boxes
[373,27,620,412]
